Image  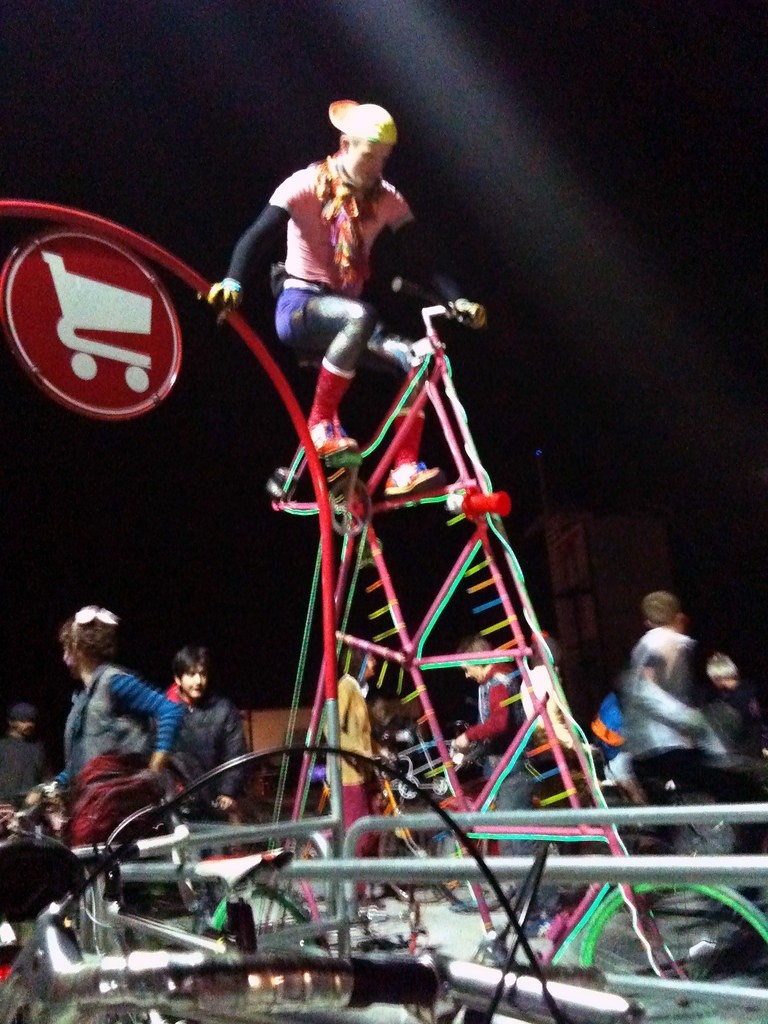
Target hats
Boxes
[329,100,397,146]
[8,703,40,722]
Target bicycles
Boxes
[0,276,767,1024]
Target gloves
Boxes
[196,277,244,307]
[450,298,487,330]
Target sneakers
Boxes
[309,419,358,459]
[385,460,445,499]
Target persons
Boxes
[197,100,487,498]
[0,591,768,920]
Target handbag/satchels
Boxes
[67,754,169,847]
